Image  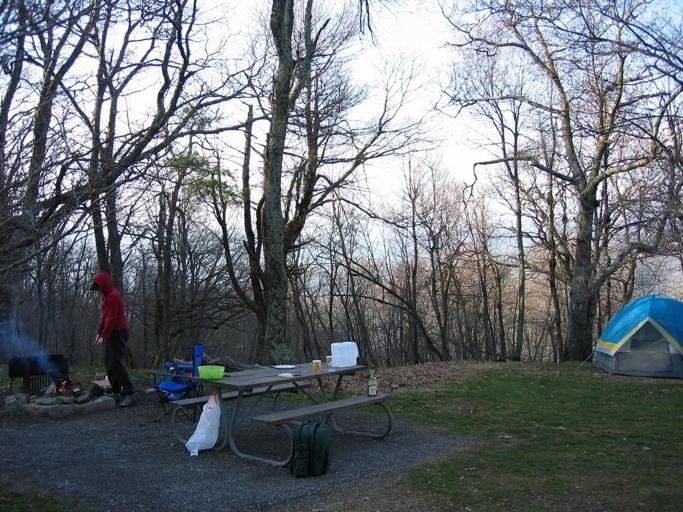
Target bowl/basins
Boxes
[197,365,226,381]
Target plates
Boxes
[273,364,296,369]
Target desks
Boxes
[199,358,367,467]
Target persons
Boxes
[88,271,137,409]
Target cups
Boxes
[325,356,335,372]
[312,359,321,374]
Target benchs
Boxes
[170,383,395,468]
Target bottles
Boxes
[366,368,378,397]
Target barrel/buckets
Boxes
[331,340,358,368]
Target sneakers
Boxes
[104,392,121,401]
[120,393,136,407]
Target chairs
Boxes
[150,342,205,424]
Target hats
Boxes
[91,283,97,290]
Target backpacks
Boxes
[289,420,331,477]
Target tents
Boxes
[591,295,683,382]
